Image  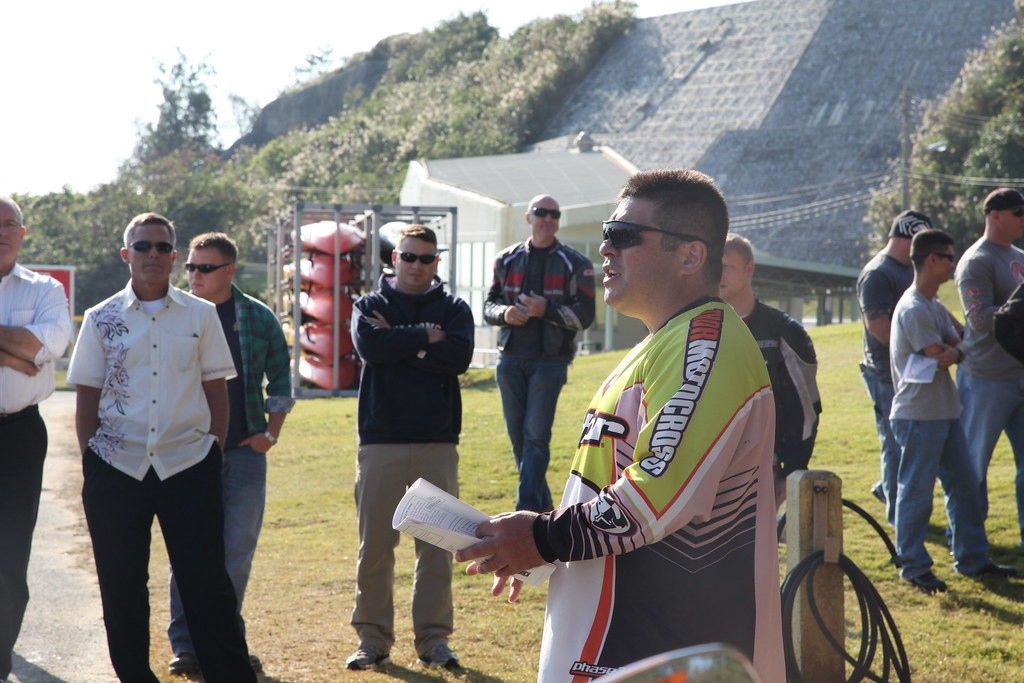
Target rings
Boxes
[523,307,528,314]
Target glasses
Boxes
[925,251,956,262]
[529,207,561,219]
[184,262,228,273]
[127,240,174,254]
[994,207,1024,216]
[395,250,437,264]
[601,220,706,249]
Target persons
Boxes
[855,187,1024,591]
[66,212,295,683]
[718,231,823,518]
[0,195,71,683]
[455,170,789,682]
[483,199,596,515]
[345,235,475,669]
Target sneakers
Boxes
[346,646,391,669]
[417,651,462,670]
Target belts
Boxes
[1,403,38,426]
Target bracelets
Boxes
[264,433,277,446]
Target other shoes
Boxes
[975,561,1016,577]
[169,653,199,671]
[908,571,948,595]
[248,653,263,670]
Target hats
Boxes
[888,210,932,237]
[983,188,1024,214]
